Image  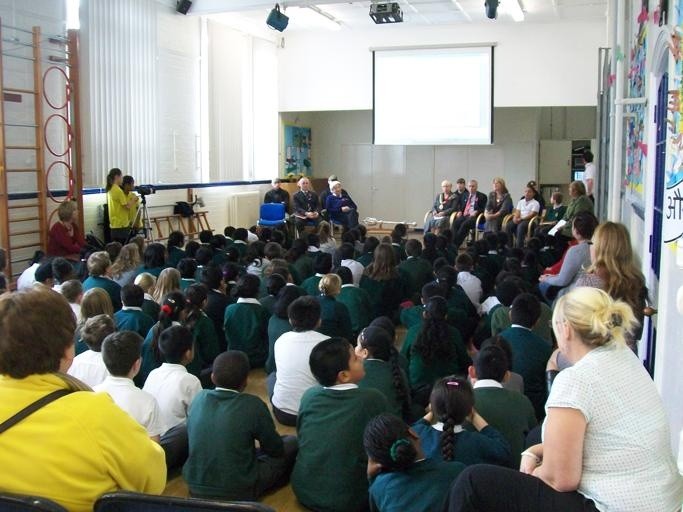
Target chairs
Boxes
[0,485,273,512]
[424,209,547,245]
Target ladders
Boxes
[143,211,216,242]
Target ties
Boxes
[467,195,473,217]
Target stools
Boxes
[287,210,347,242]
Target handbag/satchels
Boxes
[542,223,561,243]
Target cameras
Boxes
[135,185,156,195]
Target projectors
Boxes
[369,2,403,24]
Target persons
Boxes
[104,167,131,243]
[264,172,358,240]
[0,201,682,510]
[119,174,144,234]
[423,149,596,255]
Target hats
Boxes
[528,181,537,187]
[330,181,340,192]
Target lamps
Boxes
[484,0,500,19]
[266,3,289,32]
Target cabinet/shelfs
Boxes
[538,138,597,185]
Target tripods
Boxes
[125,202,154,247]
